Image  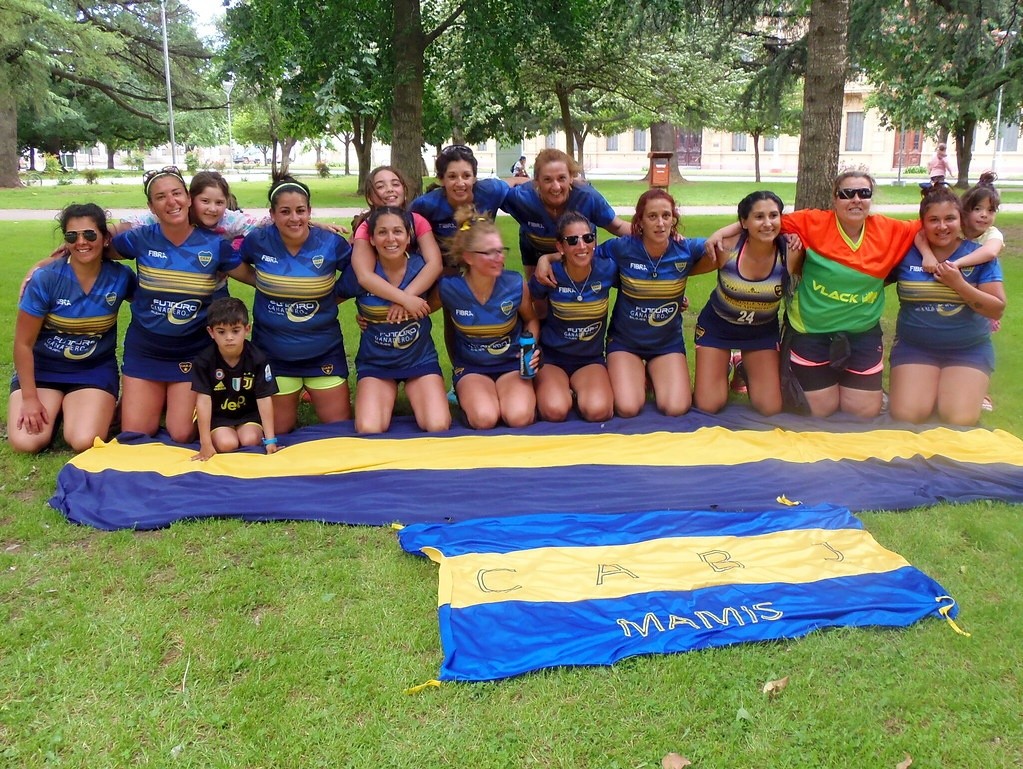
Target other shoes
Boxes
[981,396,993,412]
[447,385,460,405]
[881,391,889,414]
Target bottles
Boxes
[519,331,536,379]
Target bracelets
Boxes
[262,438,277,446]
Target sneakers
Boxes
[731,354,748,393]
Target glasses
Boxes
[143,166,182,187]
[64,229,100,243]
[441,145,470,155]
[562,233,595,245]
[836,188,872,199]
[463,247,509,259]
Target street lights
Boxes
[220,72,235,171]
[991,30,1018,174]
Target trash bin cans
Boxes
[648,151,673,188]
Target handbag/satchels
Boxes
[511,164,515,173]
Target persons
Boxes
[53,172,350,298]
[703,171,922,419]
[915,171,1004,411]
[500,148,686,320]
[535,189,802,418]
[513,156,528,177]
[409,145,589,276]
[0,203,137,452]
[356,205,540,429]
[189,298,285,461]
[529,210,689,421]
[884,175,1006,428]
[333,207,463,434]
[687,191,804,417]
[929,144,955,183]
[22,171,257,441]
[351,166,443,325]
[237,170,353,434]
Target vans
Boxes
[234,143,295,166]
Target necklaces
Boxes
[643,239,668,278]
[470,276,495,302]
[553,209,558,216]
[565,265,591,302]
